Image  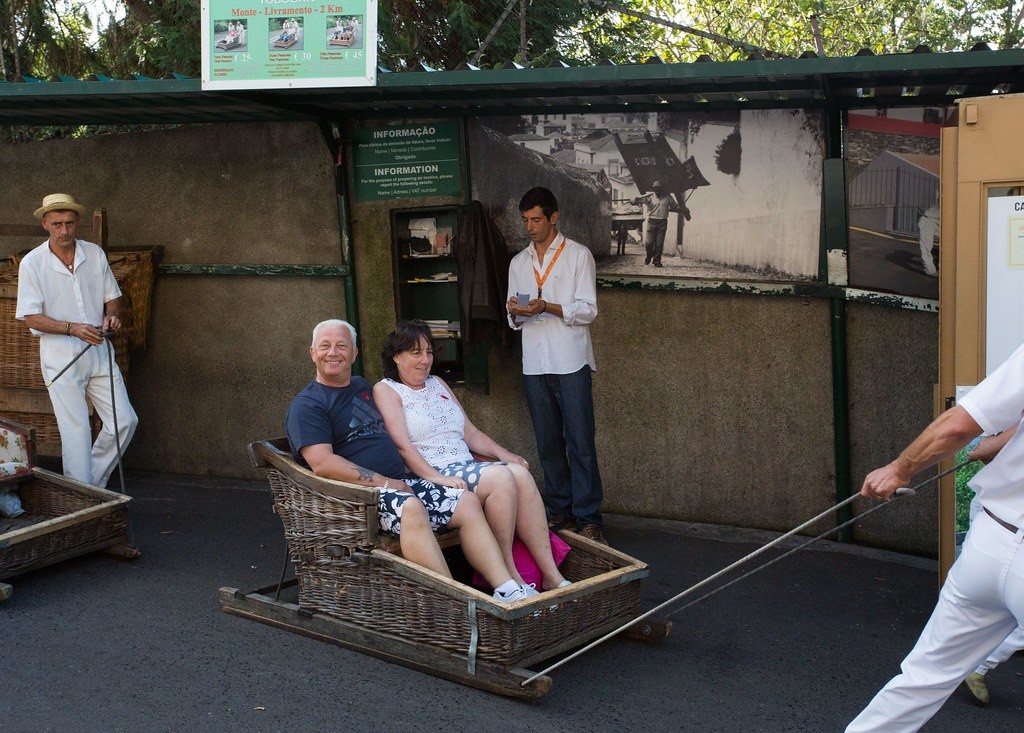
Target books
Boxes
[422,320,455,338]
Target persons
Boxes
[616,224,629,256]
[372,320,572,595]
[334,17,358,39]
[841,342,1024,733]
[284,318,527,603]
[630,180,678,267]
[280,18,298,42]
[224,21,245,46]
[15,194,138,490]
[505,187,609,547]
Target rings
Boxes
[524,462,526,463]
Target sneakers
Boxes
[549,519,578,535]
[492,588,541,616]
[521,584,558,614]
[581,524,611,559]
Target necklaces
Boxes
[51,247,74,270]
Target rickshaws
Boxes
[0,417,144,599]
[217,432,675,705]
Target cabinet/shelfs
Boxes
[389,201,490,396]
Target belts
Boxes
[983,506,1020,534]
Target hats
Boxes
[290,18,296,22]
[284,20,288,23]
[351,17,356,20]
[652,180,662,188]
[33,193,88,219]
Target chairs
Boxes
[220,437,673,699]
[0,416,142,600]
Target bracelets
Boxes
[539,300,546,314]
[66,322,72,336]
[384,477,389,488]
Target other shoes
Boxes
[966,672,991,705]
[644,257,651,264]
[555,580,584,601]
[651,261,662,267]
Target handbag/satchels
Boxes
[473,524,572,594]
